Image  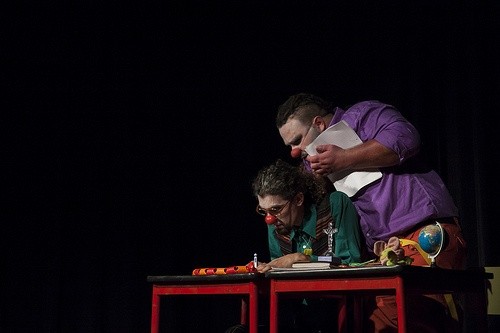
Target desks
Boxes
[146,272,268,333]
[267,265,494,332]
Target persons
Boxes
[277,92,473,333]
[251,160,375,333]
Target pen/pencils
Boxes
[254,253,259,269]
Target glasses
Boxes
[256,200,289,215]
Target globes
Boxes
[418,221,450,268]
